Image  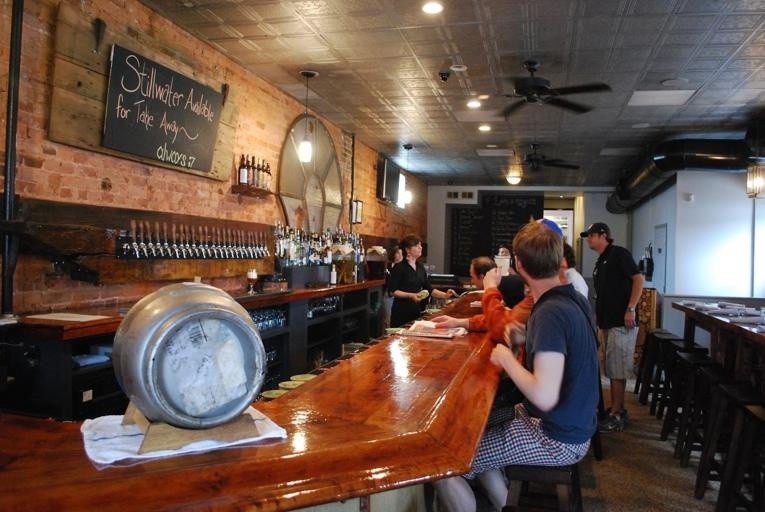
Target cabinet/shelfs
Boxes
[0,299,290,426]
[302,283,384,379]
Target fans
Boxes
[503,143,584,173]
[491,59,614,120]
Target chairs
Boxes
[506,366,606,510]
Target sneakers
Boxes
[598,408,629,433]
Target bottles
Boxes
[331,264,337,284]
[246,309,287,332]
[238,152,273,192]
[127,243,270,258]
[273,219,365,268]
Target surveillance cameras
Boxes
[439,71,450,81]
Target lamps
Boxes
[402,143,416,206]
[505,175,523,184]
[747,150,765,198]
[295,68,320,164]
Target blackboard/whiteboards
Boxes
[443,190,544,277]
[101,42,224,172]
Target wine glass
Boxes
[423,262,436,275]
[305,297,339,320]
[246,268,258,294]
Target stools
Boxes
[636,325,765,511]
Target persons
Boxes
[431,222,600,512]
[470,254,524,310]
[579,222,645,432]
[494,244,511,257]
[387,236,456,327]
[383,244,403,328]
[430,218,569,408]
[508,263,531,297]
[561,244,588,299]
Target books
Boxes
[493,255,513,277]
[402,319,468,338]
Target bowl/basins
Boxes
[111,279,266,431]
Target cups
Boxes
[266,346,276,362]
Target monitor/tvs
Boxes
[376,158,400,203]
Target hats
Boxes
[580,223,611,237]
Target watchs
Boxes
[627,307,635,312]
